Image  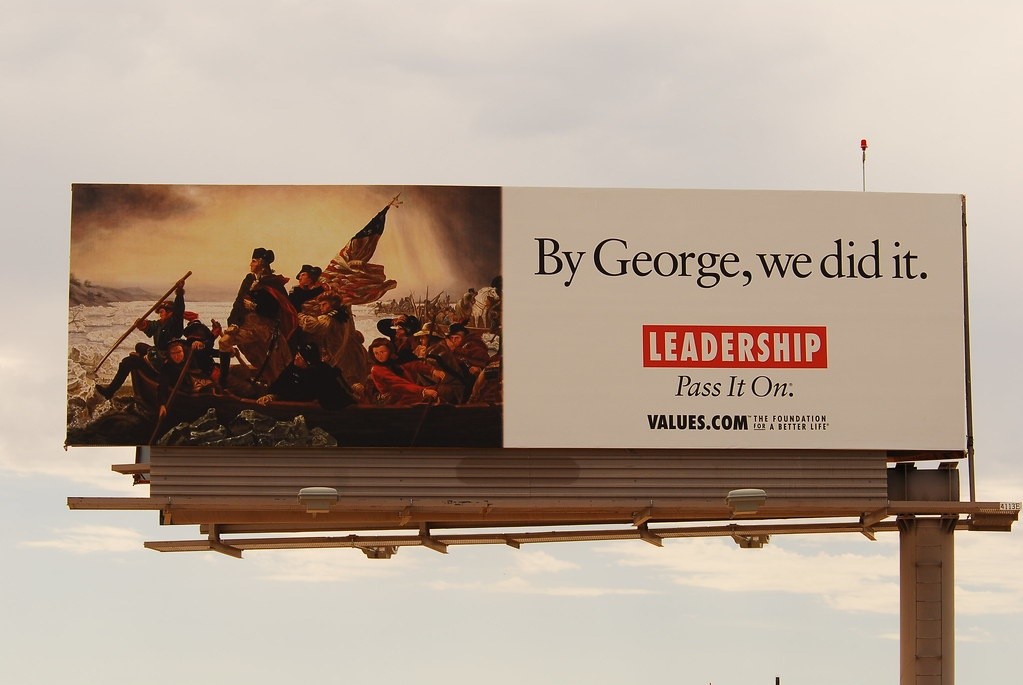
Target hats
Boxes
[414,322,446,339]
[446,321,470,338]
[166,336,190,359]
[252,248,275,268]
[395,314,421,335]
[295,265,323,284]
[155,300,175,314]
[296,342,322,369]
[459,338,491,366]
[183,319,211,338]
